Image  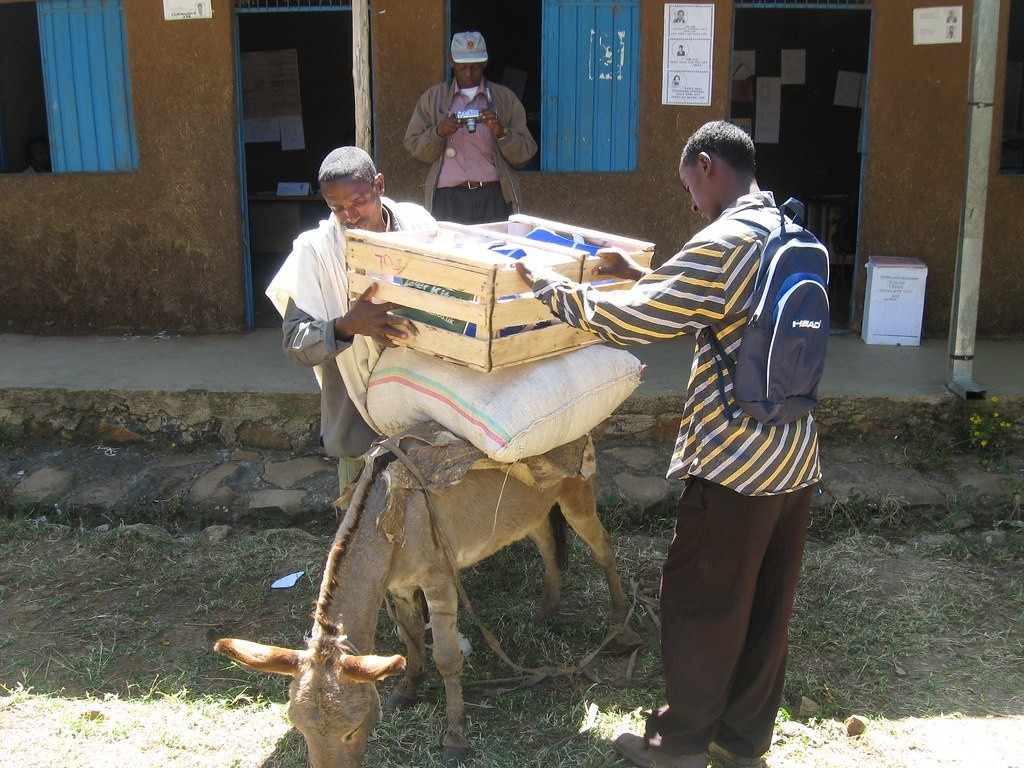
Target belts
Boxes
[455,180,500,189]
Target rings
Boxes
[495,115,498,120]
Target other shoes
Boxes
[614,732,706,768]
[397,620,474,658]
[708,742,761,768]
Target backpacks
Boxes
[700,197,830,427]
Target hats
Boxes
[451,32,488,64]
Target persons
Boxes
[403,32,539,225]
[511,120,823,768]
[264,147,471,660]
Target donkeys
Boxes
[213,421,632,768]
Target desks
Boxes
[251,195,325,268]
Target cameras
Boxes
[456,110,483,133]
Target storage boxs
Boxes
[342,213,656,373]
